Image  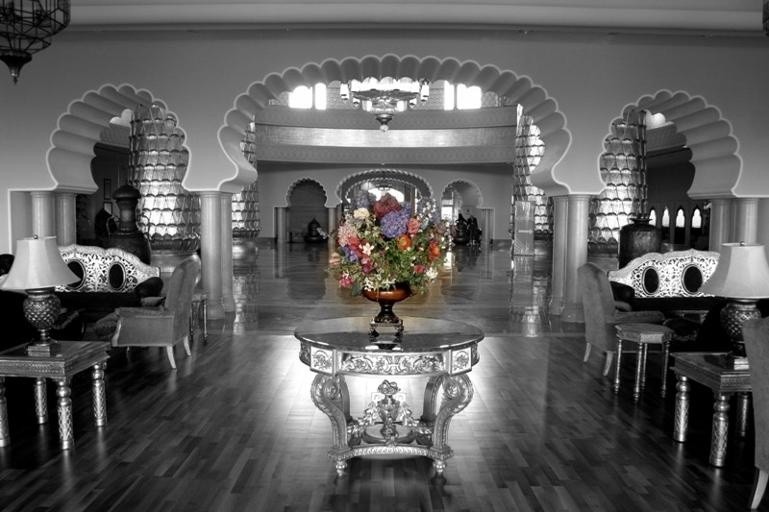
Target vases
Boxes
[360,282,412,336]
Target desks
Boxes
[290,313,486,482]
[666,349,759,471]
[0,336,111,452]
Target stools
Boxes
[610,319,677,404]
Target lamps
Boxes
[337,73,432,135]
[0,234,83,353]
[700,240,769,372]
[0,2,72,90]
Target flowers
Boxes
[332,193,446,294]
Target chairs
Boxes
[108,250,204,372]
[738,315,769,511]
[575,258,669,378]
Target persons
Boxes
[455,214,483,251]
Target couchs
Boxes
[49,242,165,329]
[607,248,769,355]
